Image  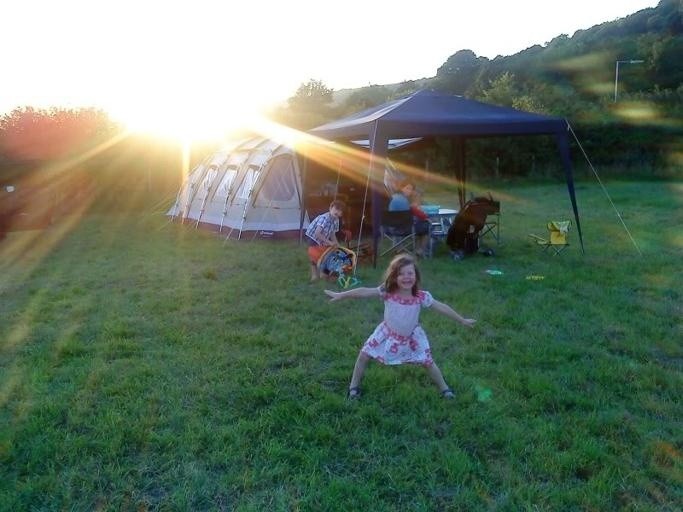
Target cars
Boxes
[0,160,65,228]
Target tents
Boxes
[164,138,423,241]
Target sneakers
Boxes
[443,389,455,398]
[350,387,361,397]
[416,249,429,260]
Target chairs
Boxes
[528,219,572,257]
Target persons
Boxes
[303,199,348,285]
[323,253,477,402]
[386,179,433,255]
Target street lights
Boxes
[614,60,645,102]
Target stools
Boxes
[378,225,417,259]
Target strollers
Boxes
[446,191,500,260]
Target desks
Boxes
[411,209,463,260]
